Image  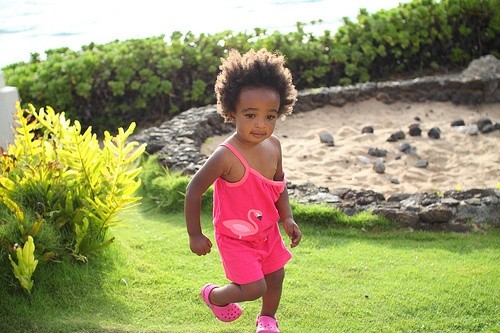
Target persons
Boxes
[185,46,302,333]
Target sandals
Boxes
[255,313,280,333]
[201,283,242,322]
